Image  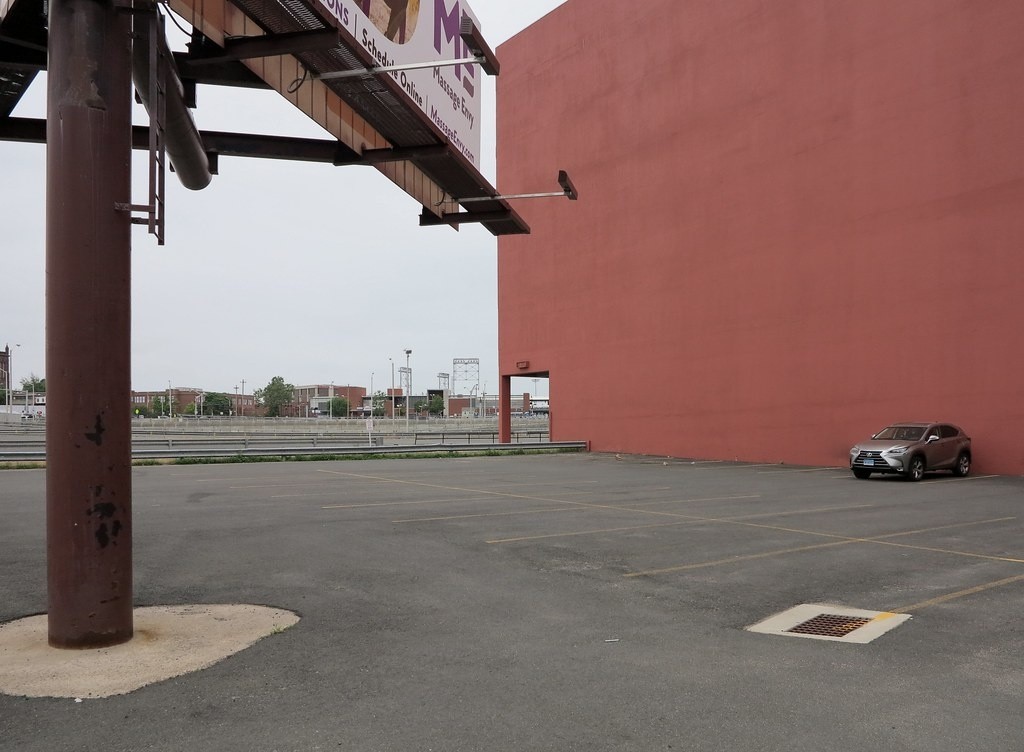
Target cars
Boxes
[849,422,972,482]
[21,413,35,425]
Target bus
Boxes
[497,412,529,418]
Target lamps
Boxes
[436,170,578,208]
[285,15,500,94]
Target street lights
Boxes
[169,380,171,422]
[371,373,374,420]
[226,397,230,416]
[330,381,334,420]
[389,358,394,433]
[31,384,34,420]
[10,343,20,414]
[397,404,402,429]
[465,383,478,411]
